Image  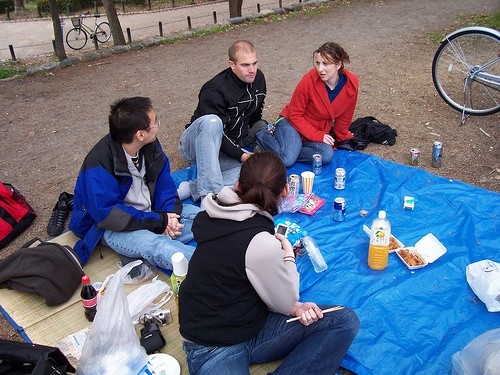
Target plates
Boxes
[139,353,181,375]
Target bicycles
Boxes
[431,26,500,125]
[66,11,112,50]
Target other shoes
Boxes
[59,192,74,209]
[47,200,70,235]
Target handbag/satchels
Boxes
[466,259,500,312]
[0,180,37,249]
[0,339,75,375]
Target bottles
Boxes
[367,209,391,271]
[171,273,187,307]
[81,275,97,322]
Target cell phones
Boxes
[275,223,288,236]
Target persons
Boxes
[177,150,360,375]
[69,96,204,272]
[240,42,359,165]
[177,40,267,204]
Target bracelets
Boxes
[283,256,296,264]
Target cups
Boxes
[171,252,189,277]
[300,171,315,195]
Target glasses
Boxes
[143,120,161,131]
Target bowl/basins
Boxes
[363,225,405,254]
[394,232,447,270]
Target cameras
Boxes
[144,310,172,326]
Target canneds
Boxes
[334,197,346,221]
[312,154,322,176]
[409,148,420,167]
[431,141,443,168]
[334,167,346,190]
[290,174,300,194]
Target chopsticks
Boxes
[285,306,345,324]
